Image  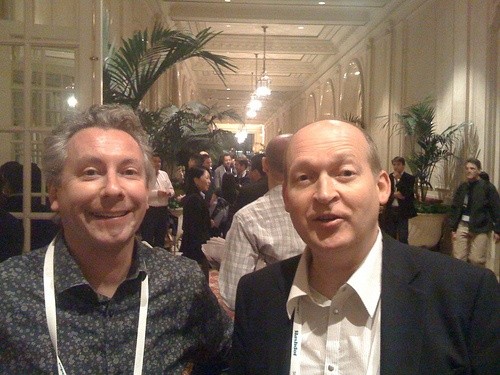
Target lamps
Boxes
[234,24,272,143]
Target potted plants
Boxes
[168,200,183,217]
[376,96,472,247]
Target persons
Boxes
[218,132,309,311]
[1,104,235,375]
[385,156,418,243]
[448,159,500,270]
[179,167,210,280]
[2,149,290,212]
[145,153,175,249]
[234,119,500,375]
